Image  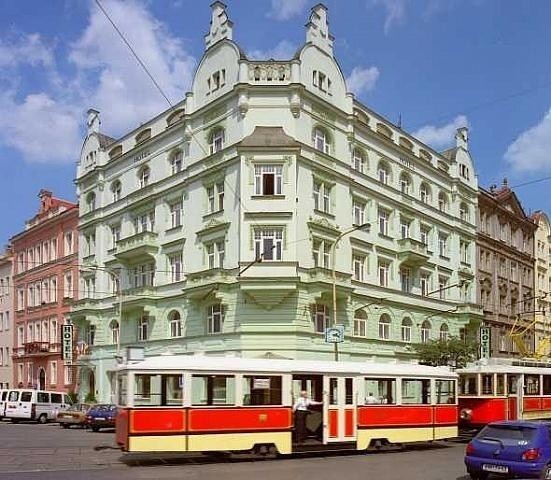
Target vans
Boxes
[0,389,10,421]
[5,389,77,423]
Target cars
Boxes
[464,420,551,480]
[85,404,125,432]
[58,401,99,428]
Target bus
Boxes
[116,356,460,461]
[457,357,551,436]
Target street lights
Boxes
[333,223,371,360]
[80,267,122,403]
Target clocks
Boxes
[325,326,345,345]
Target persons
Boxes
[292,390,323,453]
[365,391,378,407]
